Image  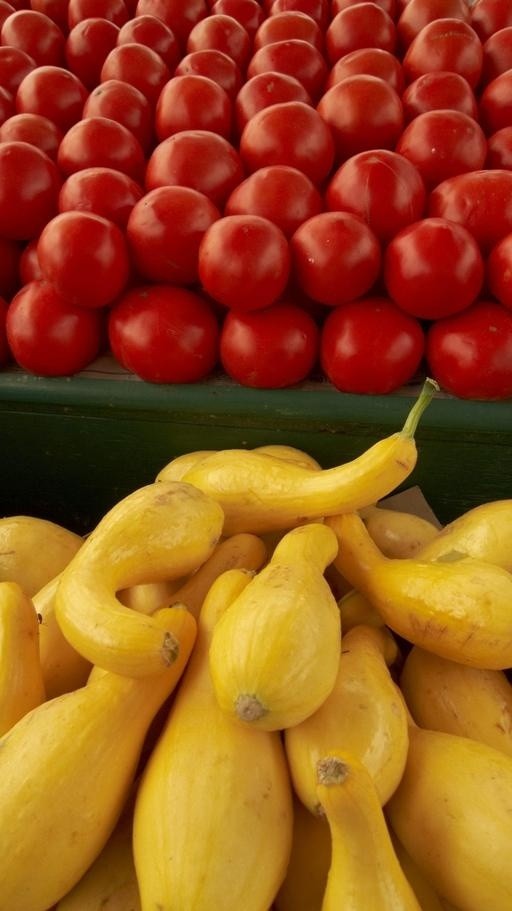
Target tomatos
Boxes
[1,0,512,400]
